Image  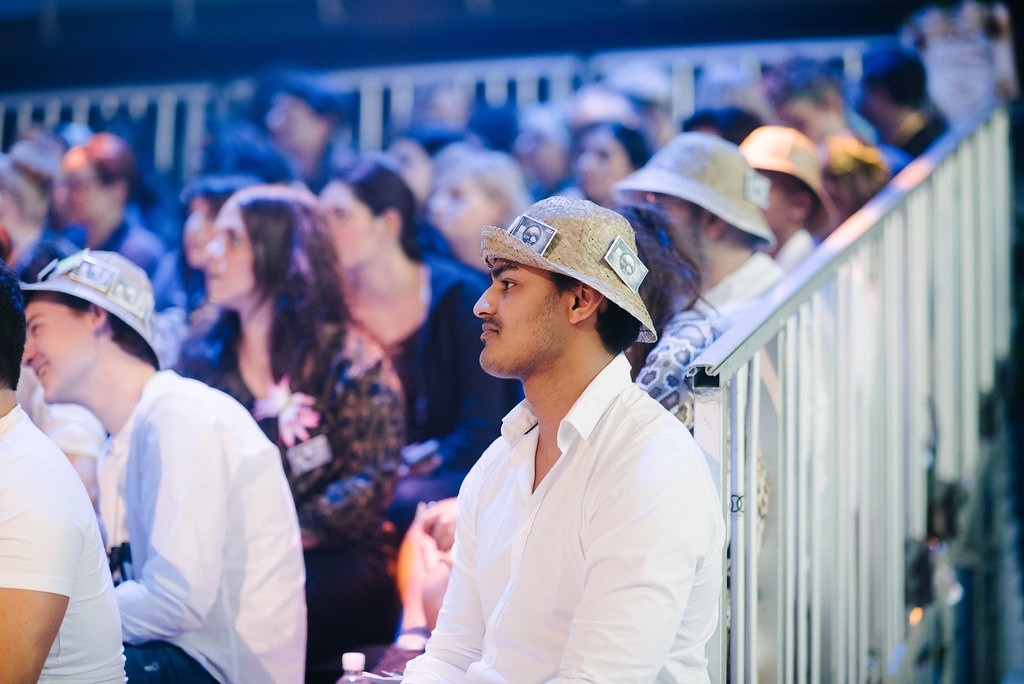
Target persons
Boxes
[371,194,771,684]
[0,255,131,684]
[17,244,310,684]
[0,44,954,539]
[157,185,413,682]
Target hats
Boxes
[738,125,835,235]
[609,131,779,254]
[18,247,162,371]
[480,195,657,344]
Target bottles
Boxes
[336,653,372,684]
